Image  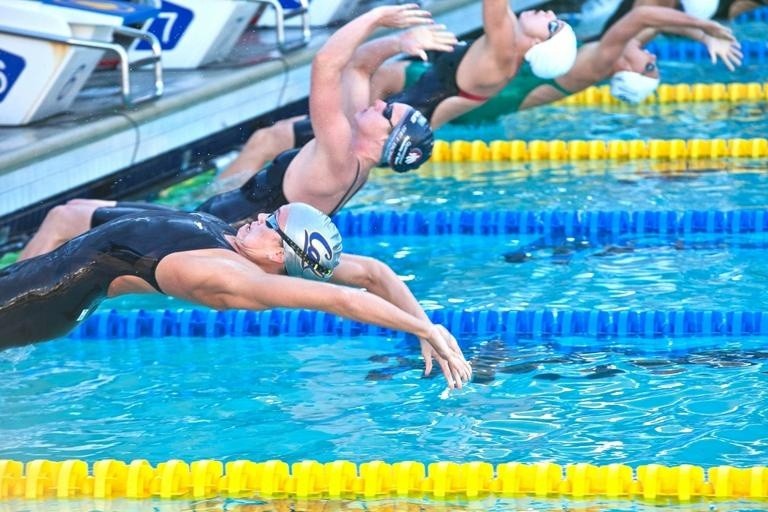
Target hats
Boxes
[280,202,343,282]
[524,22,578,79]
[381,107,436,174]
[608,71,661,109]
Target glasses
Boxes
[381,97,396,130]
[263,212,301,249]
[642,62,655,77]
[547,19,559,38]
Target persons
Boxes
[16,3,460,265]
[213,0,768,187]
[1,203,473,392]
